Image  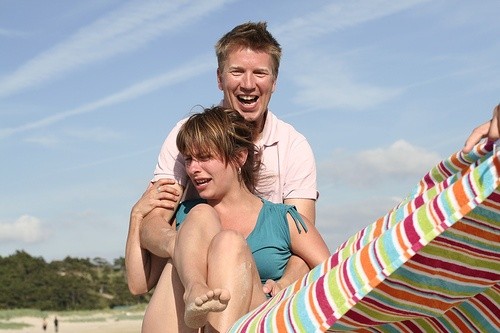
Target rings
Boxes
[157,187,160,192]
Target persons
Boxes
[43,317,47,333]
[140,23,318,298]
[125,106,332,333]
[462,102,500,154]
[53,316,59,332]
